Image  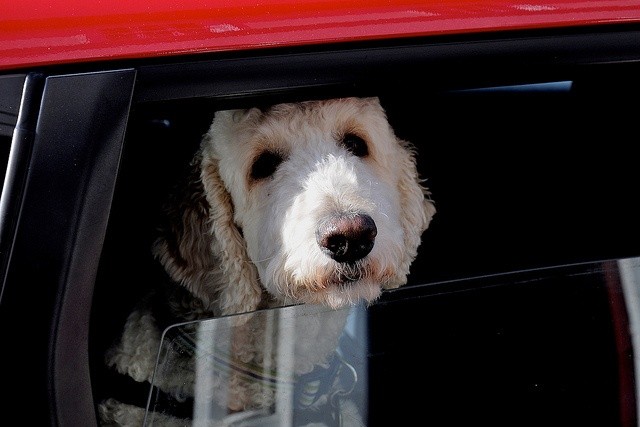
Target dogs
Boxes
[99,97,439,427]
